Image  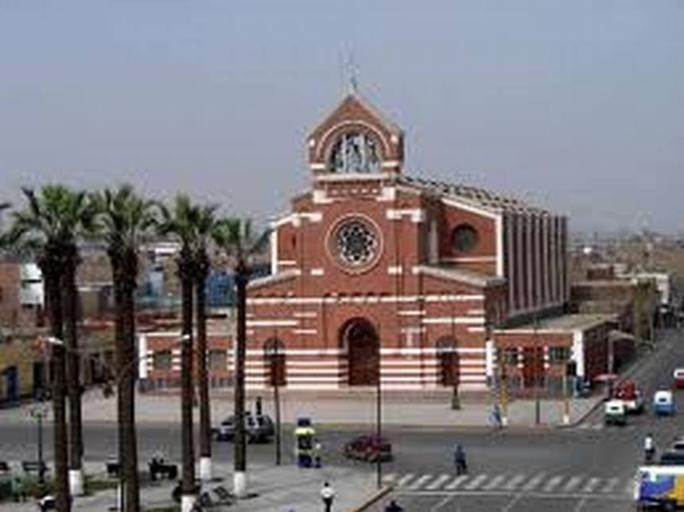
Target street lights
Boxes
[46,334,190,512]
[532,312,539,421]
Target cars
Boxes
[345,437,391,461]
[661,441,684,463]
[211,412,276,444]
[604,367,684,426]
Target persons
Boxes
[311,438,321,467]
[644,431,657,461]
[454,442,467,475]
[320,480,335,511]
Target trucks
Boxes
[632,465,684,512]
